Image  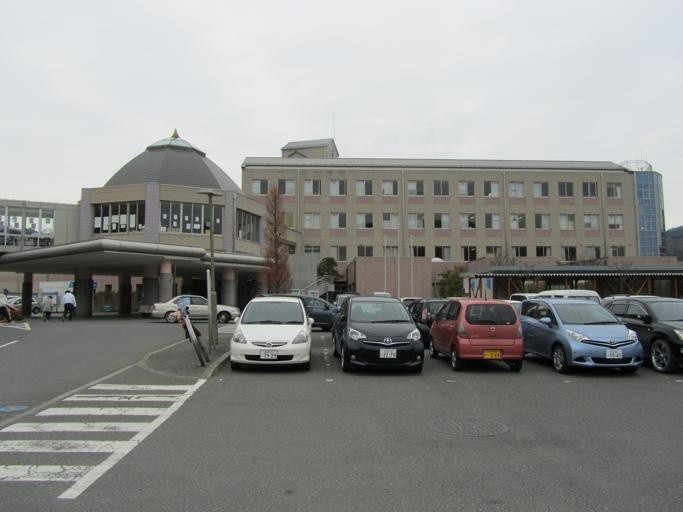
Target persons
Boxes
[41,295,54,321]
[0,289,10,323]
[60,290,75,322]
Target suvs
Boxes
[256,294,335,328]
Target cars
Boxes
[229,296,315,372]
[521,299,644,375]
[603,294,659,306]
[400,297,423,312]
[428,300,524,372]
[601,297,683,373]
[332,292,360,308]
[3,294,41,315]
[148,294,242,324]
[408,299,449,349]
[331,296,424,374]
[372,291,392,298]
[509,292,543,301]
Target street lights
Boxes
[197,189,223,346]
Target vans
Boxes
[540,289,602,302]
